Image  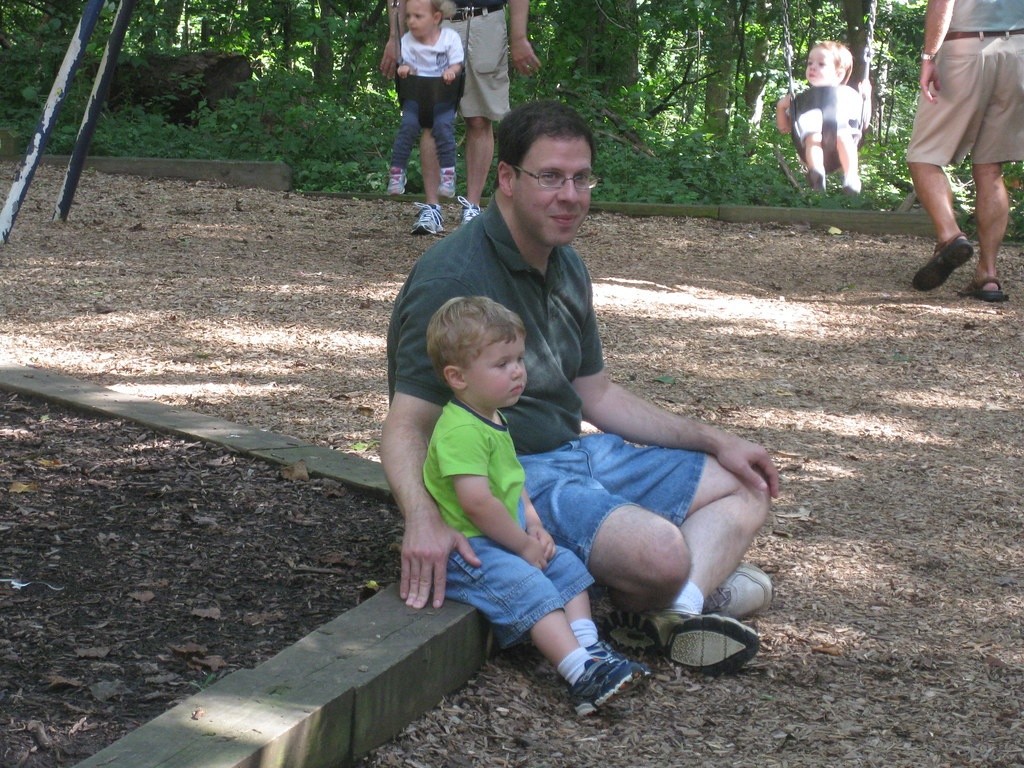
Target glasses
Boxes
[510,164,601,190]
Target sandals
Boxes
[971,278,1004,302]
[913,234,974,292]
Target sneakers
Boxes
[410,202,445,235]
[702,562,773,621]
[602,607,760,677]
[456,194,480,225]
[386,166,407,196]
[563,657,644,715]
[436,166,456,199]
[585,638,651,677]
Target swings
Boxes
[389,0,475,129]
[780,0,880,175]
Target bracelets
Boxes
[921,50,936,61]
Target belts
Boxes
[445,3,503,21]
[944,30,1024,40]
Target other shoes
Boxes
[809,168,826,193]
[844,179,861,195]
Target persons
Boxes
[379,101,780,675]
[423,296,652,717]
[776,41,872,194]
[906,0,1024,303]
[380,0,541,235]
